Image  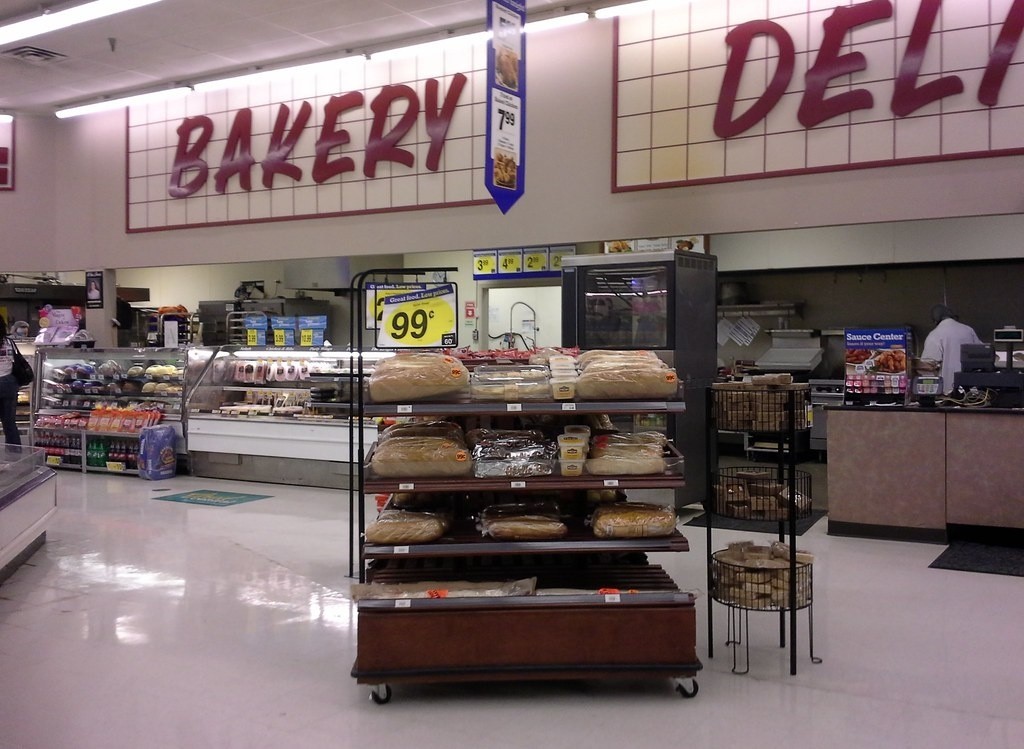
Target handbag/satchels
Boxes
[8,338,34,386]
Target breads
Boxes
[714,541,814,608]
[367,353,677,545]
[713,374,810,431]
[48,362,181,393]
[713,471,811,519]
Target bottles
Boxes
[34,434,140,469]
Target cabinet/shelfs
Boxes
[186,344,396,491]
[30,348,229,475]
[32,426,140,475]
[350,401,704,706]
[706,387,822,675]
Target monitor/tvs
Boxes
[960,344,996,373]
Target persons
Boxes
[0,315,23,453]
[918,305,985,395]
[88,281,100,299]
[10,320,29,338]
